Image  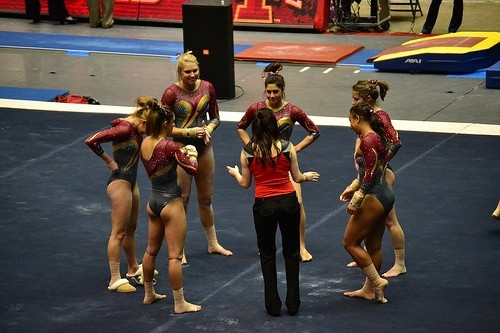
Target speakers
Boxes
[181,0,235,99]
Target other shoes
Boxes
[287,310,298,316]
[33,17,41,23]
[92,19,101,28]
[102,20,114,28]
[267,312,281,317]
[59,17,79,24]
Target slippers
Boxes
[126,264,159,279]
[108,279,136,293]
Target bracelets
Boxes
[304,173,308,181]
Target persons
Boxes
[227,108,320,315]
[24,0,79,24]
[87,0,115,28]
[235,62,320,263]
[159,50,233,267]
[346,79,408,278]
[339,103,395,303]
[85,97,158,293]
[141,99,203,313]
[420,0,463,34]
